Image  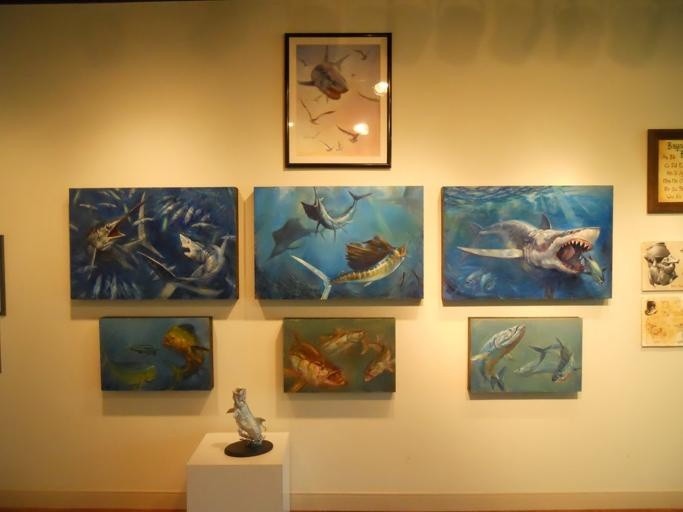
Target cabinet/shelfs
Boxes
[186,430,291,512]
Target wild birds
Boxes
[300,100,360,151]
[353,47,371,61]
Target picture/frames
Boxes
[645,125,683,216]
[282,29,394,171]
